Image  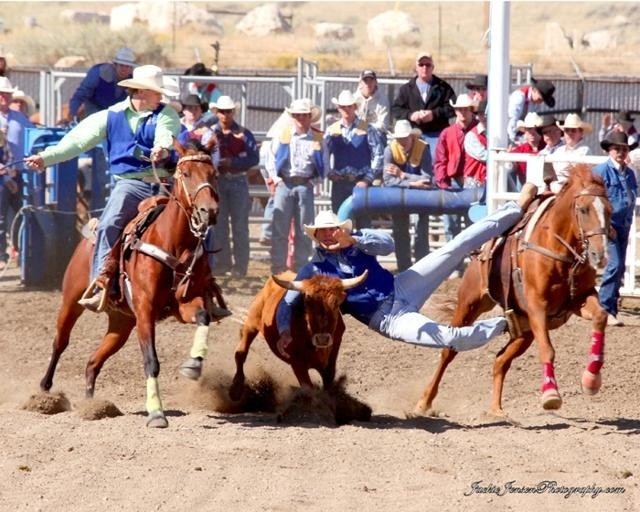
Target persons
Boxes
[272,182,541,359]
[175,50,487,277]
[507,78,640,326]
[22,66,182,312]
[0,49,137,268]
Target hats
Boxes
[515,107,640,152]
[208,94,241,116]
[116,63,181,98]
[183,62,218,77]
[448,72,489,110]
[330,88,364,110]
[528,76,558,109]
[110,47,142,68]
[284,97,323,124]
[415,51,434,62]
[358,68,378,82]
[0,76,36,117]
[302,209,354,245]
[178,94,209,114]
[387,118,423,141]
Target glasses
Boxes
[543,127,562,135]
[417,62,432,68]
[563,127,579,134]
[217,109,233,114]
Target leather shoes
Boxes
[77,288,107,310]
[515,181,539,210]
[211,299,234,319]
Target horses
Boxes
[412,161,613,419]
[39,134,222,430]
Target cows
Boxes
[229,269,370,401]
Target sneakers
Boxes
[605,312,625,326]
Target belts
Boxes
[280,174,312,185]
[136,175,169,185]
[340,175,364,182]
